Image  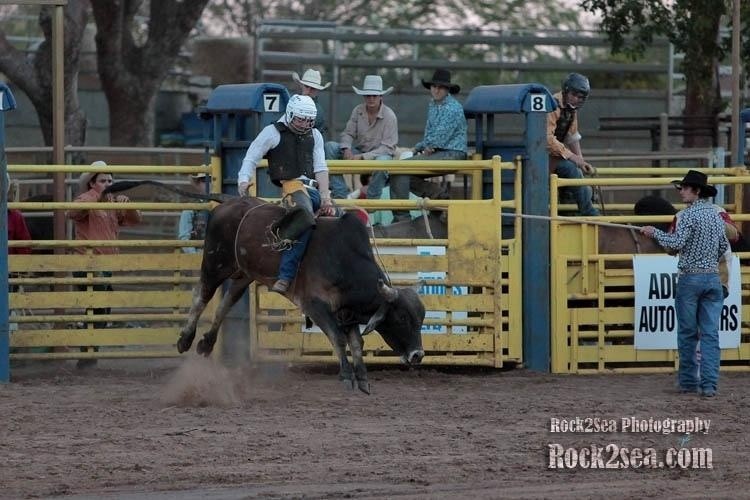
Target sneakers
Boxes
[663,384,717,399]
[270,279,290,294]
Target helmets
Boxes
[559,72,591,111]
[284,94,317,134]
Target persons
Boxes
[6,171,31,330]
[664,203,740,378]
[640,170,729,398]
[64,160,143,368]
[324,75,399,213]
[292,68,331,133]
[238,93,343,292]
[178,164,211,290]
[546,73,601,216]
[389,68,468,222]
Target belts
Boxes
[680,269,720,274]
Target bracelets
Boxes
[320,190,331,200]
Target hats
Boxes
[78,161,115,194]
[671,169,718,199]
[292,68,333,92]
[421,70,461,95]
[351,74,395,98]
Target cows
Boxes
[101,181,425,395]
[599,223,664,345]
[366,215,447,239]
